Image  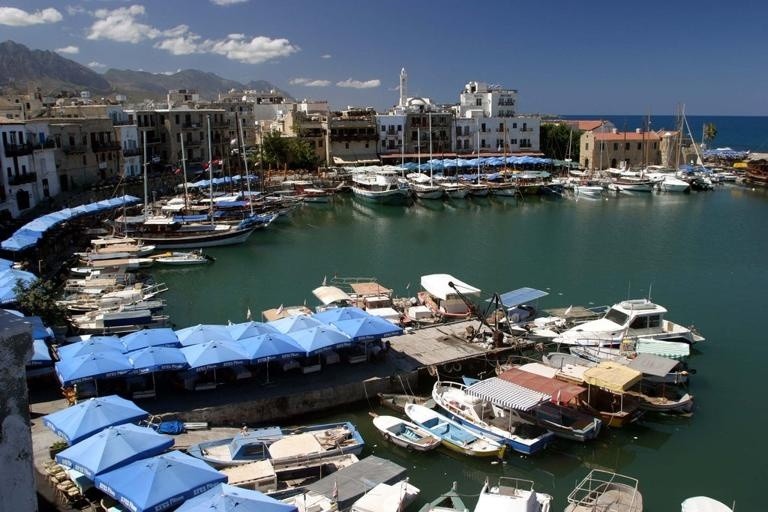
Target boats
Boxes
[473,476,554,512]
[53,234,208,338]
[261,274,480,328]
[681,496,734,512]
[418,479,470,512]
[563,468,644,512]
[143,418,420,512]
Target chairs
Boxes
[60,386,77,406]
[38,459,120,512]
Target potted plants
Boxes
[49,442,70,459]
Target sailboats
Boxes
[111,195,305,250]
